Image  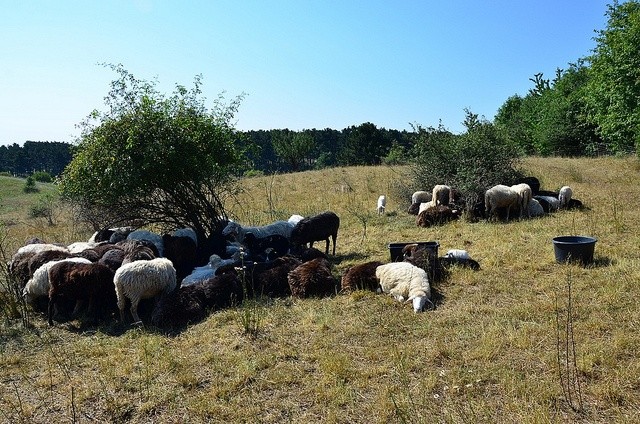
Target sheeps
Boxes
[407,177,583,228]
[340,244,424,295]
[376,195,386,217]
[6,211,340,336]
[375,262,435,314]
[445,249,472,261]
